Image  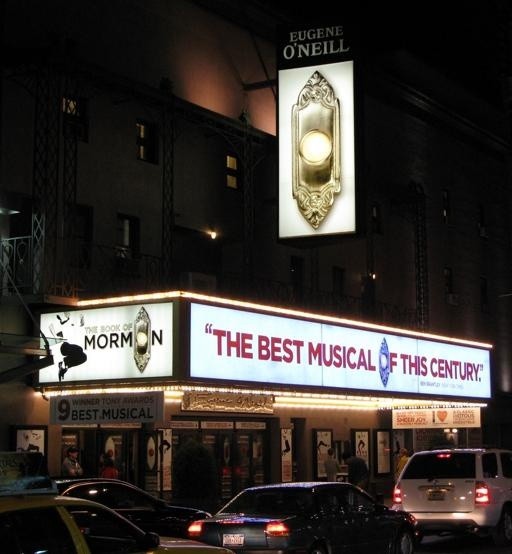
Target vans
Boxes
[391,446,511,546]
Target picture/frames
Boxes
[375,429,393,476]
[352,427,371,474]
[314,428,334,478]
[10,423,50,460]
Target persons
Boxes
[100,458,118,479]
[397,448,410,481]
[61,447,83,478]
[324,448,341,482]
[342,450,369,489]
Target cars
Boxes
[187,481,424,554]
[0,477,236,554]
